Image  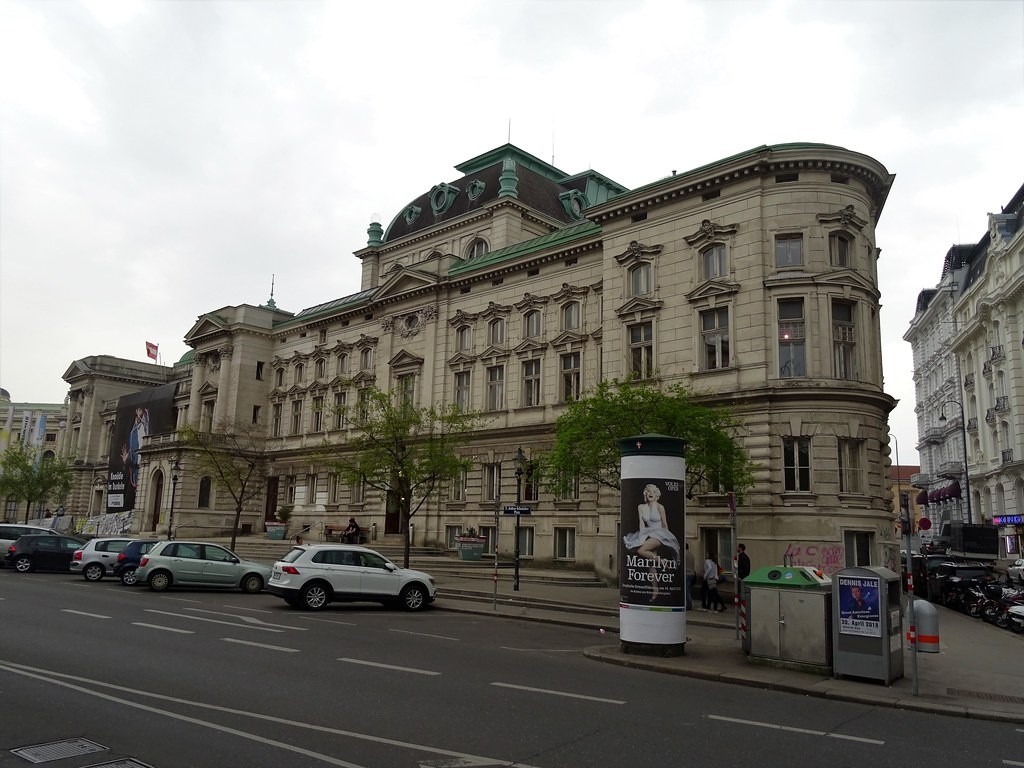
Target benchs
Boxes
[324,524,370,543]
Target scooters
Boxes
[945,569,1024,636]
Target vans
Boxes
[900,549,918,576]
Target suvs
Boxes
[933,561,996,591]
[912,554,957,601]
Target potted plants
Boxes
[454,524,488,561]
[264,504,294,540]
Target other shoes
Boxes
[706,605,711,609]
[712,609,718,613]
[718,605,727,612]
[697,606,707,612]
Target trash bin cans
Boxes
[830,565,906,686]
[743,564,832,672]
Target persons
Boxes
[0,518,12,524]
[624,484,680,602]
[686,543,750,613]
[343,517,360,544]
[44,505,65,518]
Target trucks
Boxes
[940,524,1000,564]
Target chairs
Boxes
[335,552,345,565]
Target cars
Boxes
[266,544,438,612]
[0,523,82,563]
[1004,559,1024,585]
[113,539,221,587]
[69,537,159,582]
[135,540,272,595]
[3,534,90,573]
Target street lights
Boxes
[937,400,973,525]
[512,446,530,591]
[166,462,182,541]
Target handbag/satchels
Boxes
[708,579,717,590]
[690,569,696,586]
